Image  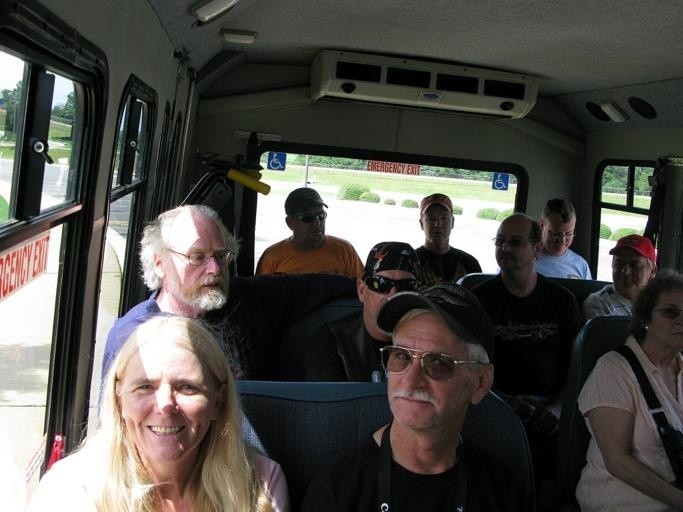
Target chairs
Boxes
[460,272,616,316]
[228,273,366,375]
[548,314,634,512]
[231,375,535,512]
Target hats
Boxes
[375,279,491,341]
[609,234,656,265]
[361,241,421,281]
[420,193,453,215]
[284,188,329,215]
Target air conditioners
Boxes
[308,50,542,121]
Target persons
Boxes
[575,269,683,512]
[294,241,423,383]
[533,198,592,281]
[99,206,239,420]
[27,314,289,511]
[468,214,581,466]
[415,194,482,291]
[582,234,658,320]
[254,188,365,279]
[300,281,533,511]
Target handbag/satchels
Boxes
[616,344,683,490]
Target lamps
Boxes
[220,30,258,47]
[192,1,240,21]
[598,102,627,123]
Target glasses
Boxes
[167,248,233,266]
[379,344,489,381]
[365,274,422,294]
[492,238,538,247]
[652,303,679,318]
[550,229,576,240]
[295,211,327,223]
